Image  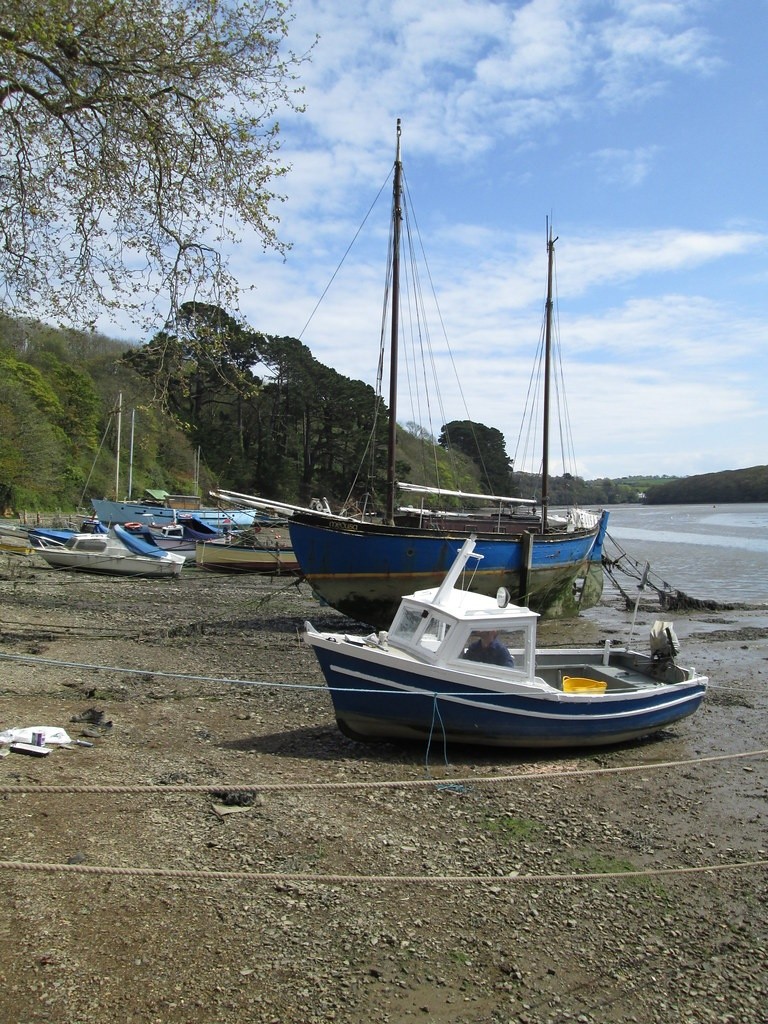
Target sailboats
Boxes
[204,115,609,620]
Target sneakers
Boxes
[83,721,114,737]
[72,707,104,722]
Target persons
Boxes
[464,630,515,668]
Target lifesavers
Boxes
[124,521,142,529]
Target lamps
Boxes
[497,587,510,608]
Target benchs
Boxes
[588,665,665,689]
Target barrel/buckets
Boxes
[562,675,607,692]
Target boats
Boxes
[257,512,289,527]
[195,541,300,573]
[25,523,198,577]
[90,396,256,534]
[302,531,711,751]
[0,526,36,555]
